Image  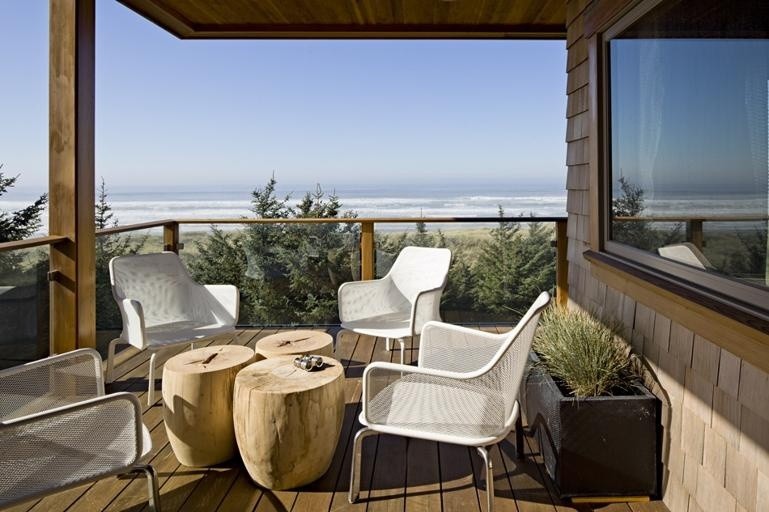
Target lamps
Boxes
[107,250,239,407]
[658,242,716,270]
[348,292,551,512]
[0,347,161,512]
[335,246,452,377]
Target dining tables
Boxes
[518,298,660,504]
